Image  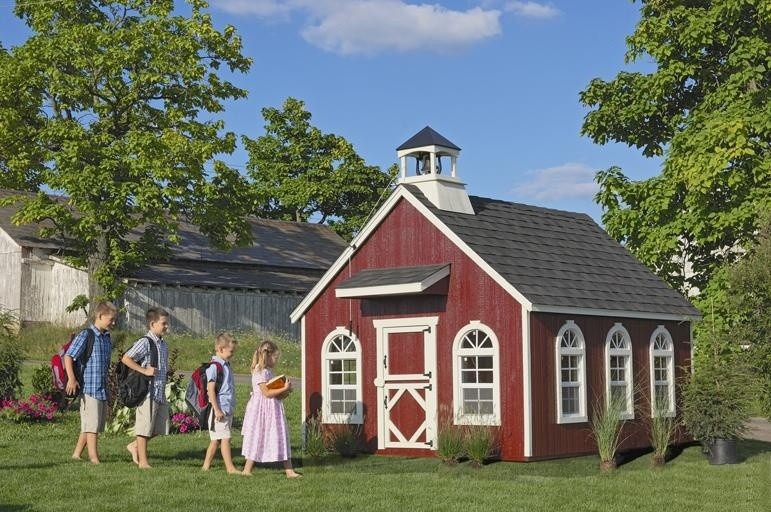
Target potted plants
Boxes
[587,383,637,471]
[642,374,678,465]
[684,326,760,464]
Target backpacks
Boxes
[185,361,223,430]
[51,328,95,397]
[115,336,158,408]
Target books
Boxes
[266,374,293,400]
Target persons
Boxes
[240,341,305,479]
[201,331,243,474]
[121,308,171,468]
[64,301,119,466]
